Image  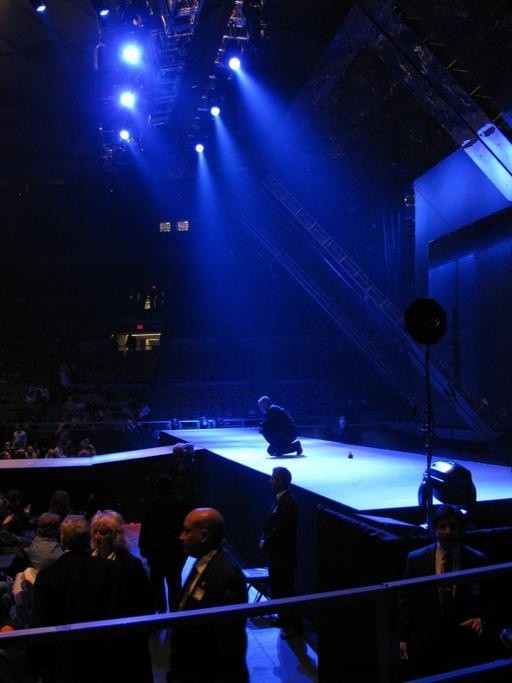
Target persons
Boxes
[127,333,136,350]
[0,398,197,682]
[164,506,249,681]
[257,395,303,458]
[334,416,357,443]
[255,466,304,638]
[397,504,497,679]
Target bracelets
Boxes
[479,617,487,627]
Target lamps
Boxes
[89,1,269,169]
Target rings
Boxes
[400,648,403,654]
